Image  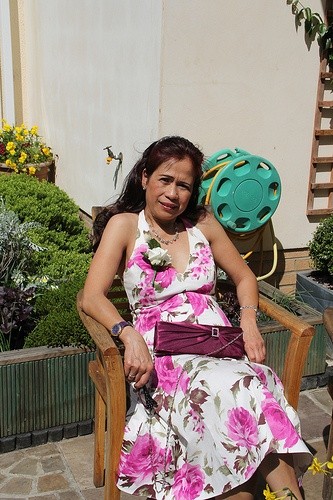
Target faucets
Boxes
[102,144,123,165]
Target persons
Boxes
[82,136,313,500]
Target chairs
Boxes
[320,307,333,500]
[76,288,316,500]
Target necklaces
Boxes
[145,219,180,245]
[239,305,258,317]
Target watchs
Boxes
[111,321,134,342]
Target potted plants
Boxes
[294,212,333,318]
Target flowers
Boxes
[0,122,58,177]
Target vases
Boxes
[0,160,53,183]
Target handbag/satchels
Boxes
[153,320,244,360]
